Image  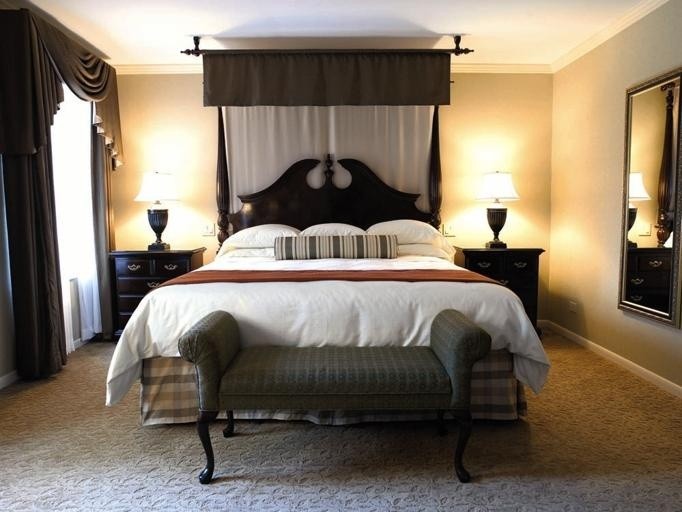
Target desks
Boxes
[625,245,672,313]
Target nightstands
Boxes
[454,245,545,337]
[108,245,206,346]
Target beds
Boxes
[105,153,550,427]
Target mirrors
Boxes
[617,66,682,332]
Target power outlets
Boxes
[568,301,577,313]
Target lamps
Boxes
[474,171,520,248]
[133,174,180,250]
[628,171,651,249]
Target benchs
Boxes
[179,309,491,484]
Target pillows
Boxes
[212,219,457,262]
